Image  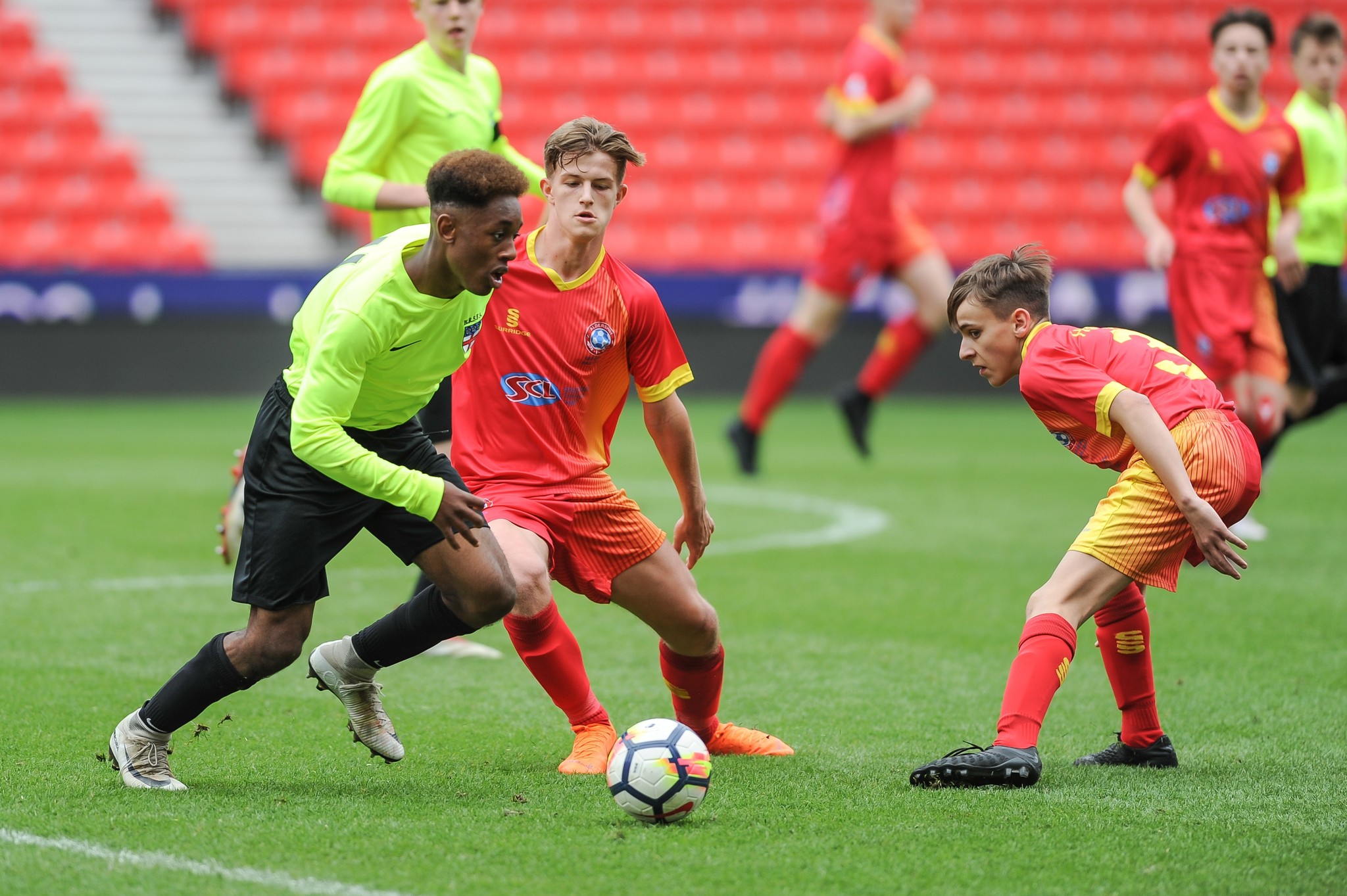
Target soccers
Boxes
[606,718,712,824]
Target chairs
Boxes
[0,0,1347,268]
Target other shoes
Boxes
[729,420,756,474]
[1228,515,1268,543]
[843,389,874,456]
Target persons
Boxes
[909,232,1262,789]
[452,115,795,776]
[720,0,954,476]
[108,149,531,792]
[1265,14,1347,439]
[1125,7,1306,545]
[319,0,548,659]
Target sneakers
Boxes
[216,445,254,564]
[1071,732,1178,770]
[559,715,617,775]
[702,721,795,757]
[108,712,188,792]
[908,740,1043,788]
[305,638,404,764]
